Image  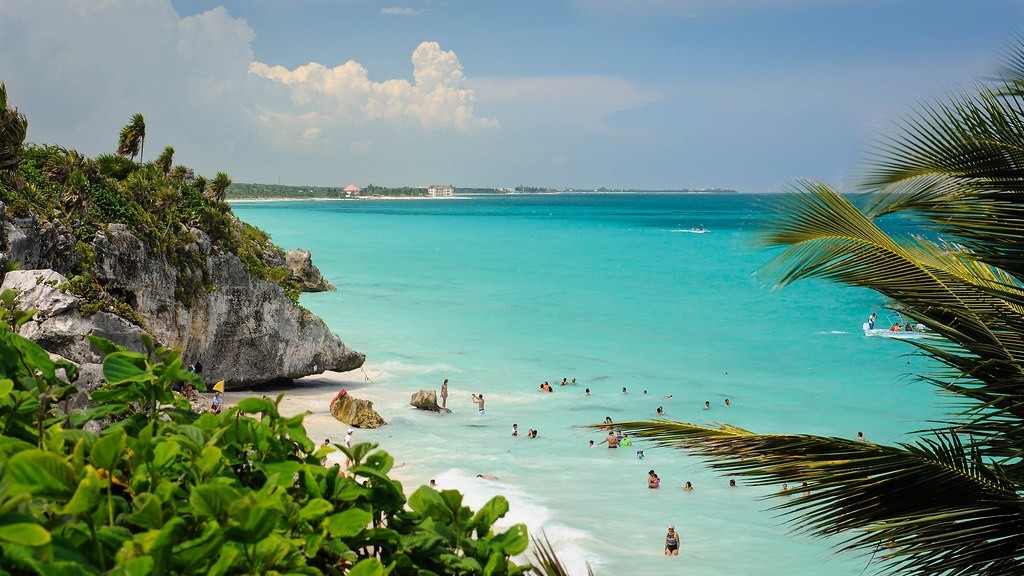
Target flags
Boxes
[213,381,224,394]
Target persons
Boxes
[477,474,498,480]
[648,470,660,488]
[665,524,680,556]
[636,450,644,459]
[209,392,222,415]
[512,424,518,435]
[622,387,628,394]
[868,313,877,329]
[657,406,664,415]
[585,389,590,396]
[597,416,632,448]
[560,378,567,386]
[588,440,594,447]
[684,482,693,491]
[572,378,576,383]
[643,390,647,394]
[471,394,485,415]
[539,382,553,392]
[890,322,926,332]
[724,399,730,406]
[320,439,330,466]
[343,428,354,467]
[730,480,736,488]
[856,432,868,441]
[430,480,437,487]
[666,395,672,399]
[441,379,448,408]
[703,401,709,410]
[527,429,537,438]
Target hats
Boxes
[667,524,674,529]
[347,428,352,434]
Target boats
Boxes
[866,329,922,338]
